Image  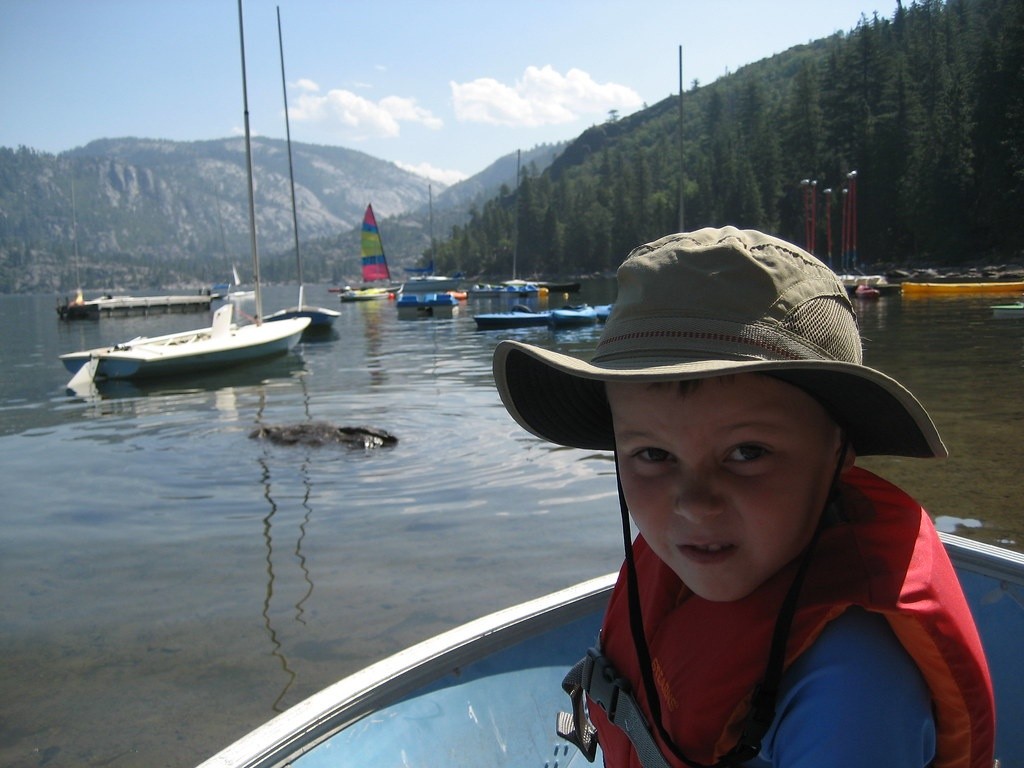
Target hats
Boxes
[492,225,947,459]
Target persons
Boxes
[493,226,997,768]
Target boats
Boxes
[57,283,231,320]
[856,284,881,300]
[901,290,1024,302]
[835,270,901,298]
[329,149,612,330]
[900,279,1024,293]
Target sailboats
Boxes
[251,4,343,331]
[57,1,311,399]
